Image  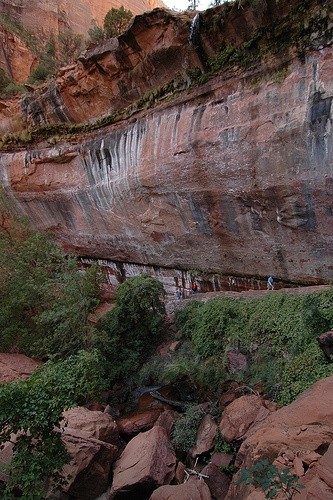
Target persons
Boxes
[175,288,182,300]
[191,282,197,294]
[267,275,274,290]
